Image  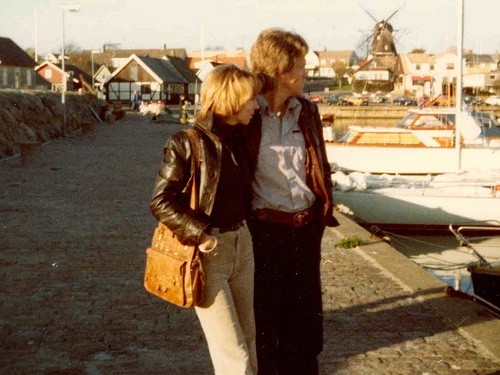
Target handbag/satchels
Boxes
[143,128,208,308]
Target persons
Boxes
[131,91,142,112]
[73,68,83,92]
[177,94,188,125]
[246,28,341,375]
[150,63,262,375]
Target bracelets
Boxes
[199,235,218,253]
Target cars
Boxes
[326,94,368,106]
[463,96,500,107]
[392,96,411,106]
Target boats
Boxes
[323,110,500,175]
[448,219,500,316]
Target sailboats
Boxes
[332,0,500,236]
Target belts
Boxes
[204,222,244,236]
[257,206,315,228]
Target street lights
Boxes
[61,7,79,134]
[92,49,100,90]
